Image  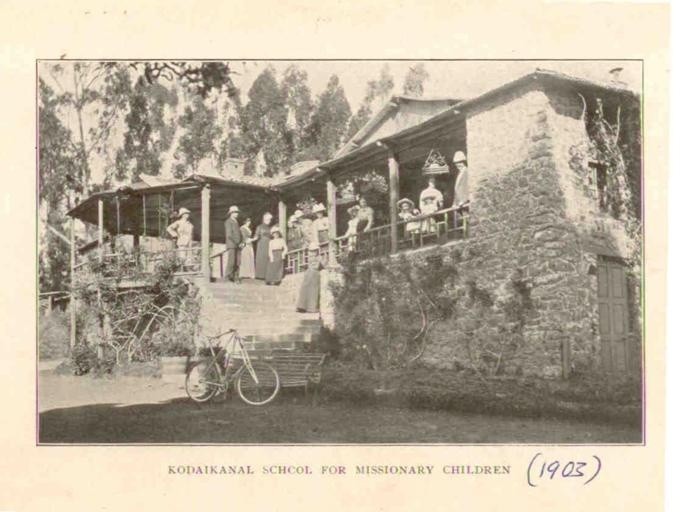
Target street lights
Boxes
[67,173,83,203]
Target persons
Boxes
[419,193,438,234]
[341,203,359,252]
[294,208,312,270]
[166,205,195,273]
[311,202,328,265]
[238,215,259,280]
[263,226,287,282]
[254,210,272,279]
[287,215,303,273]
[450,149,470,222]
[222,204,241,281]
[396,196,421,234]
[417,176,446,210]
[355,194,375,251]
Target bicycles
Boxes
[185,329,280,406]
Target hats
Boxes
[452,150,467,163]
[346,205,361,214]
[176,207,191,218]
[308,241,320,251]
[312,203,327,213]
[396,198,415,209]
[289,209,305,222]
[227,205,240,215]
[270,226,282,236]
